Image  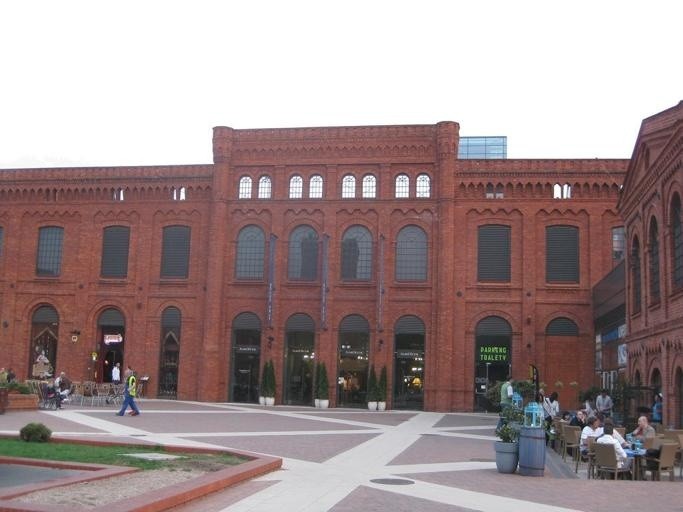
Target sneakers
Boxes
[115,411,136,416]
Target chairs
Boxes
[26,380,143,410]
[554,417,683,481]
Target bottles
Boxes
[635,439,640,452]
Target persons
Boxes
[538,388,632,481]
[45,371,72,409]
[632,416,659,471]
[111,362,121,385]
[0,367,8,383]
[495,375,515,434]
[115,369,140,416]
[7,368,16,384]
[124,366,133,383]
[650,392,663,423]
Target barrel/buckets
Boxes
[517,426,547,476]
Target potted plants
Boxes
[318,362,329,409]
[377,365,386,412]
[365,365,378,411]
[265,359,276,406]
[495,424,519,473]
[259,362,268,405]
[314,363,320,408]
